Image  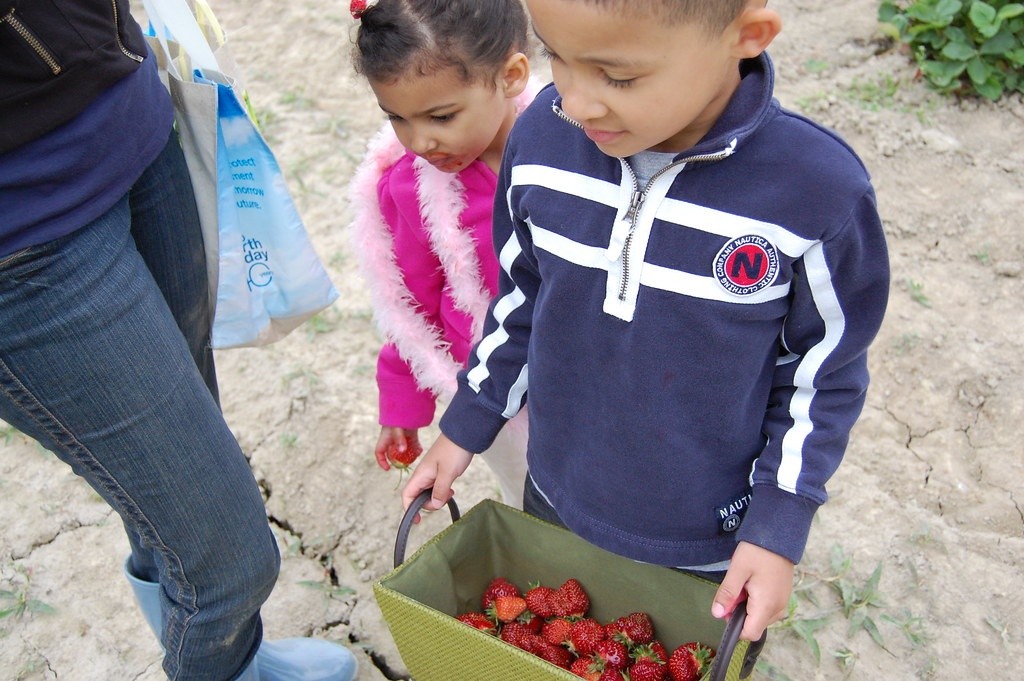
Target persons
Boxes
[352,0,540,512]
[0,0,360,681]
[399,0,895,681]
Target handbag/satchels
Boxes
[139,0,340,350]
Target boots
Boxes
[121,550,361,681]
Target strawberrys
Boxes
[456,578,715,681]
[387,436,416,468]
